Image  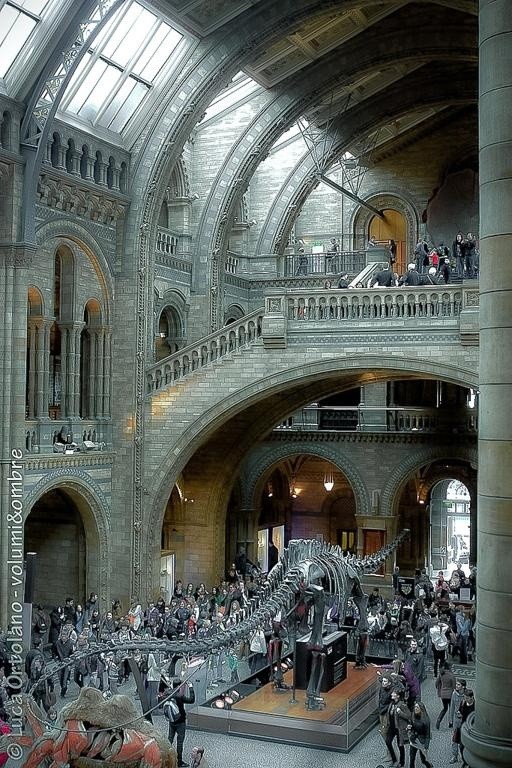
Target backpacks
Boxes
[163,697,181,721]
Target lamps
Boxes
[290,485,304,500]
[321,459,336,493]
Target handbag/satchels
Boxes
[46,692,56,706]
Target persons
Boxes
[1,534,477,768]
[297,239,308,274]
[329,232,476,315]
